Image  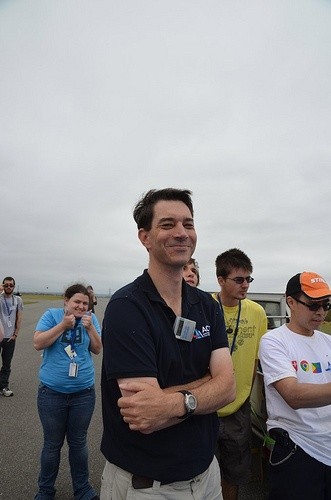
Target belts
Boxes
[132,474,175,489]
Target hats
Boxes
[285,272,331,300]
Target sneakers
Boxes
[0,387,14,397]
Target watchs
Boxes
[177,390,197,419]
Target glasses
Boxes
[226,276,254,284]
[4,284,14,288]
[294,298,331,312]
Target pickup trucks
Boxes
[202,291,292,444]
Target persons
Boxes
[183,258,200,287]
[0,277,24,397]
[210,248,268,500]
[87,285,97,313]
[258,271,331,500]
[100,187,237,500]
[34,284,103,500]
